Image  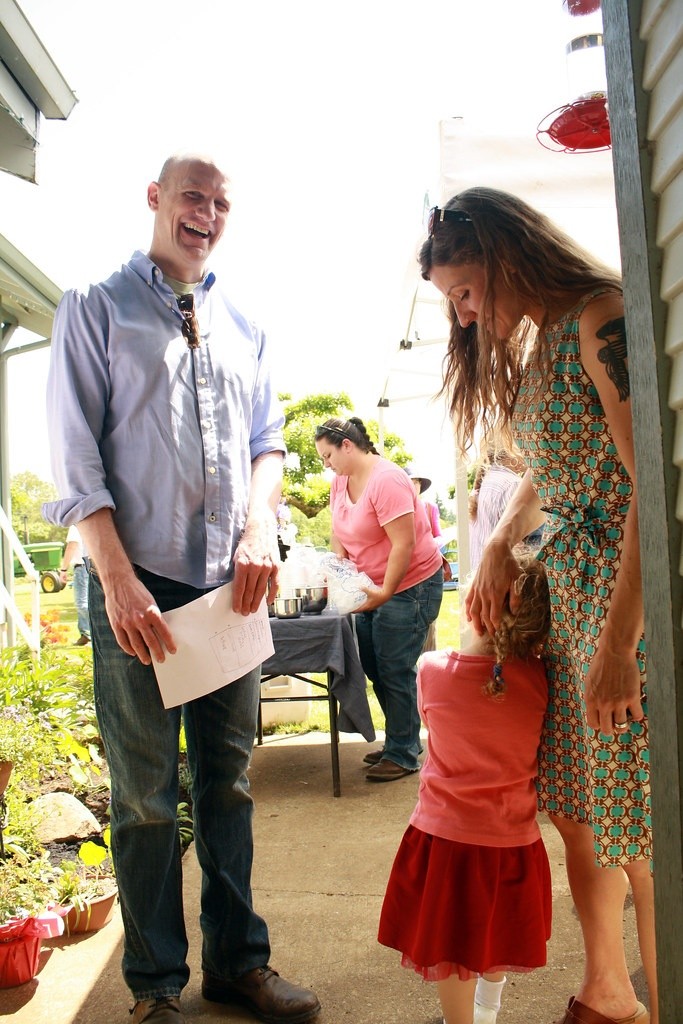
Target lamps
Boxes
[535,0,612,155]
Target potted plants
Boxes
[0,642,195,987]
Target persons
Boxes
[419,188,659,1024]
[469,431,530,583]
[314,418,444,781]
[378,559,553,1024]
[41,156,321,1024]
[404,466,452,650]
[61,569,66,572]
[60,523,94,647]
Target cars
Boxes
[443,562,459,590]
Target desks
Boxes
[257,608,376,798]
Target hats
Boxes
[408,469,433,494]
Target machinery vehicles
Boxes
[12,514,67,594]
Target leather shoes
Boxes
[363,749,382,764]
[205,969,323,1024]
[557,993,650,1023]
[366,758,408,782]
[124,995,192,1023]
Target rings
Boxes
[614,722,627,728]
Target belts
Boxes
[73,564,84,568]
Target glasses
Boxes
[428,206,477,235]
[175,293,201,350]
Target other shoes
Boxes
[75,636,90,646]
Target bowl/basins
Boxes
[294,586,328,615]
[274,597,302,619]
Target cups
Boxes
[301,537,310,544]
